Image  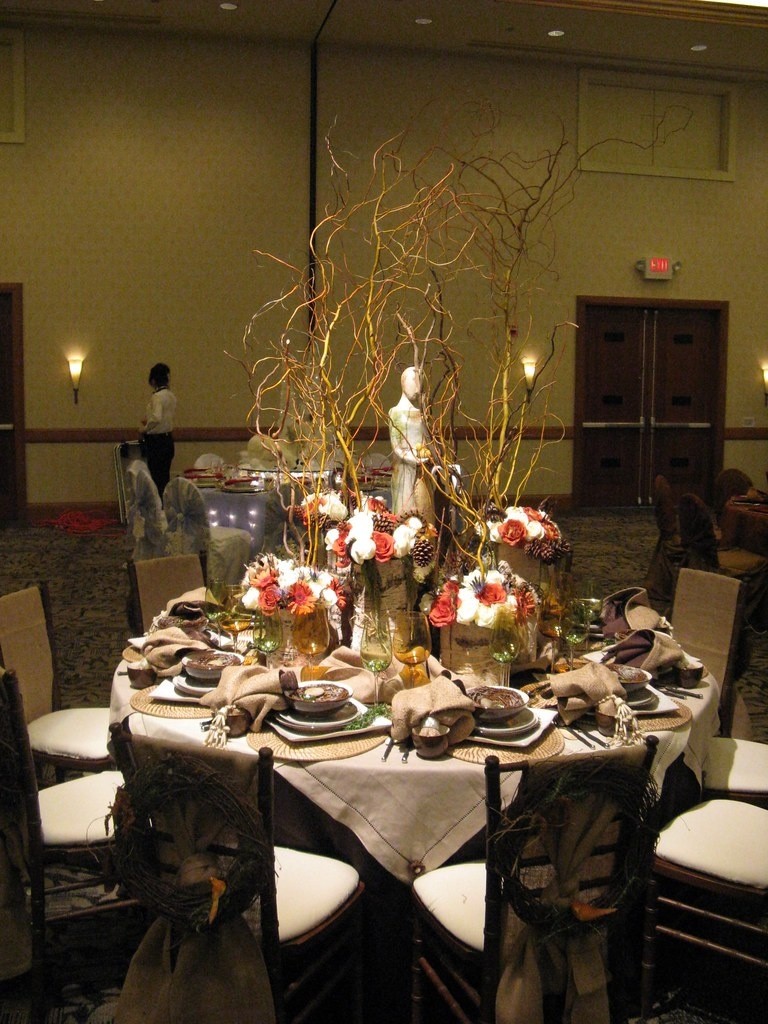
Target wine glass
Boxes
[201,577,254,659]
[361,611,433,707]
[489,575,604,691]
[255,596,331,682]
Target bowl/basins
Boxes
[282,681,353,714]
[602,660,652,697]
[463,684,530,724]
[181,649,244,684]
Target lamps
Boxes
[67,359,83,405]
[523,361,536,402]
[635,257,681,280]
[761,368,768,407]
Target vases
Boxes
[351,558,412,630]
[439,622,511,690]
[266,610,324,668]
[497,545,540,582]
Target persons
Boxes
[388,366,436,530]
[139,362,177,511]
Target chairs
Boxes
[163,476,254,603]
[121,460,168,568]
[678,492,768,627]
[642,474,722,601]
[194,451,391,469]
[714,468,753,528]
[261,482,322,569]
[1,550,768,1024]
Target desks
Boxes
[721,494,768,635]
[109,623,722,1024]
[198,485,273,561]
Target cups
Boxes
[672,661,703,690]
[411,724,450,760]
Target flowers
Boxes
[325,508,440,614]
[240,552,346,610]
[292,488,390,532]
[474,504,572,566]
[419,560,541,631]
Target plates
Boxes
[257,699,393,741]
[172,673,219,695]
[598,682,680,715]
[459,709,559,747]
